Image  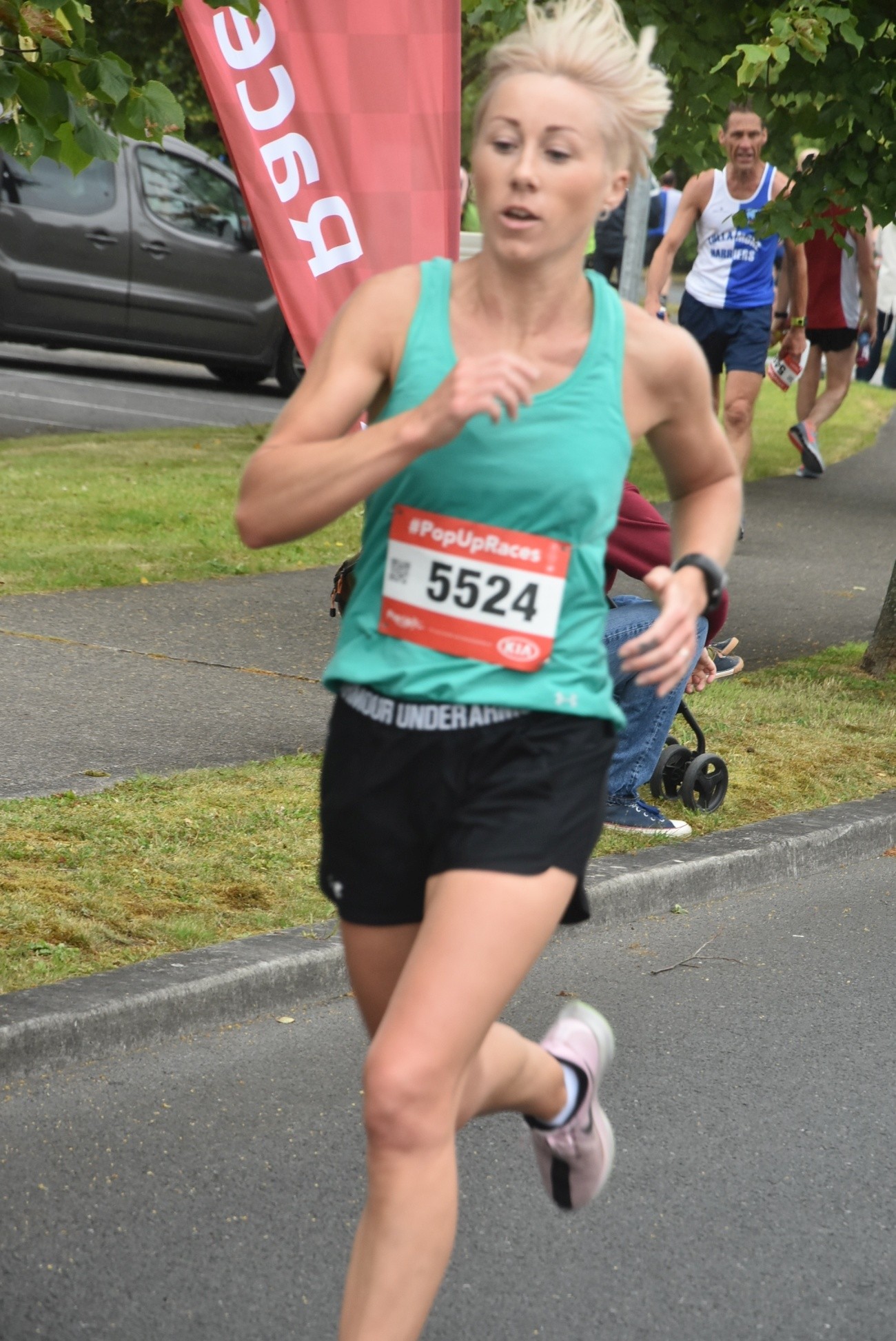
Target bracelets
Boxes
[775,312,788,318]
[790,316,805,328]
[672,553,725,612]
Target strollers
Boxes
[602,598,731,814]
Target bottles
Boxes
[855,329,871,367]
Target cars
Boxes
[0,113,307,393]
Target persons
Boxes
[237,1,746,1341]
[583,167,682,322]
[599,481,743,838]
[765,173,896,478]
[643,103,808,472]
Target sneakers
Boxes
[523,995,615,1211]
[600,788,691,838]
[787,422,825,473]
[708,637,739,655]
[707,646,744,681]
[794,464,816,478]
